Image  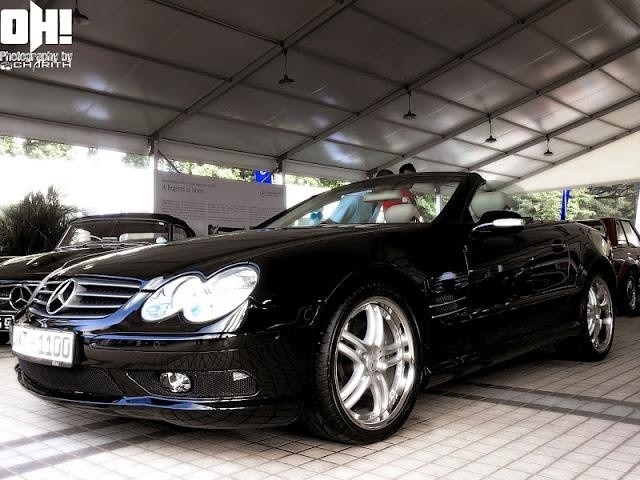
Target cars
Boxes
[1,213,195,344]
[600,218,640,317]
[9,172,615,446]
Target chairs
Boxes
[385,204,421,223]
[470,192,509,221]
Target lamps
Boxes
[68,1,91,25]
[403,88,418,121]
[484,115,496,144]
[275,39,295,88]
[544,136,553,156]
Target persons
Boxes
[324,169,394,224]
[383,162,418,223]
